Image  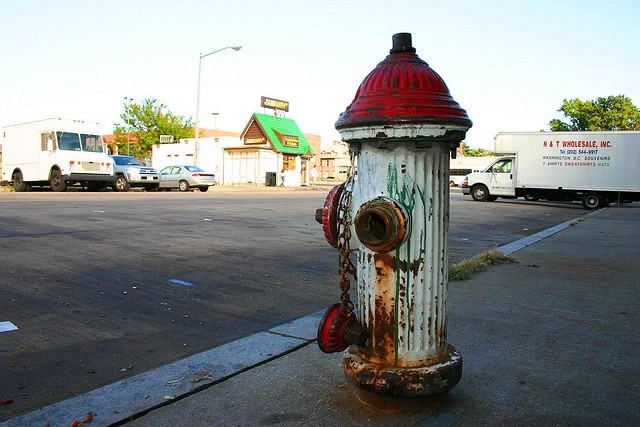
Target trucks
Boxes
[2,117,118,191]
[461,130,640,210]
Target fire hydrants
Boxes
[315,32,473,409]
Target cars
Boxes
[155,165,216,191]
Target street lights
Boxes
[124,96,134,155]
[194,44,242,164]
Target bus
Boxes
[449,165,490,188]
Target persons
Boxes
[309,164,319,189]
[280,168,286,187]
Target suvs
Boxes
[81,154,162,191]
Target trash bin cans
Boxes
[266,172,276,186]
